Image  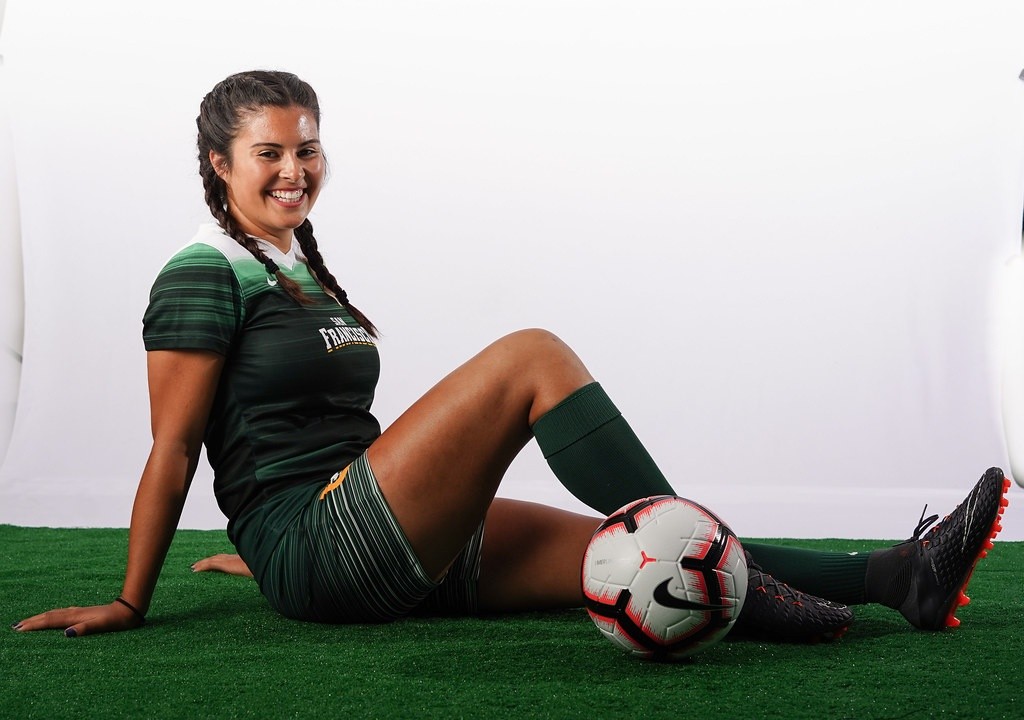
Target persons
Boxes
[10,71,1012,639]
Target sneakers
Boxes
[723,549,855,643]
[890,467,1011,631]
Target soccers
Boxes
[580,494,748,662]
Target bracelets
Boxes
[115,597,144,620]
